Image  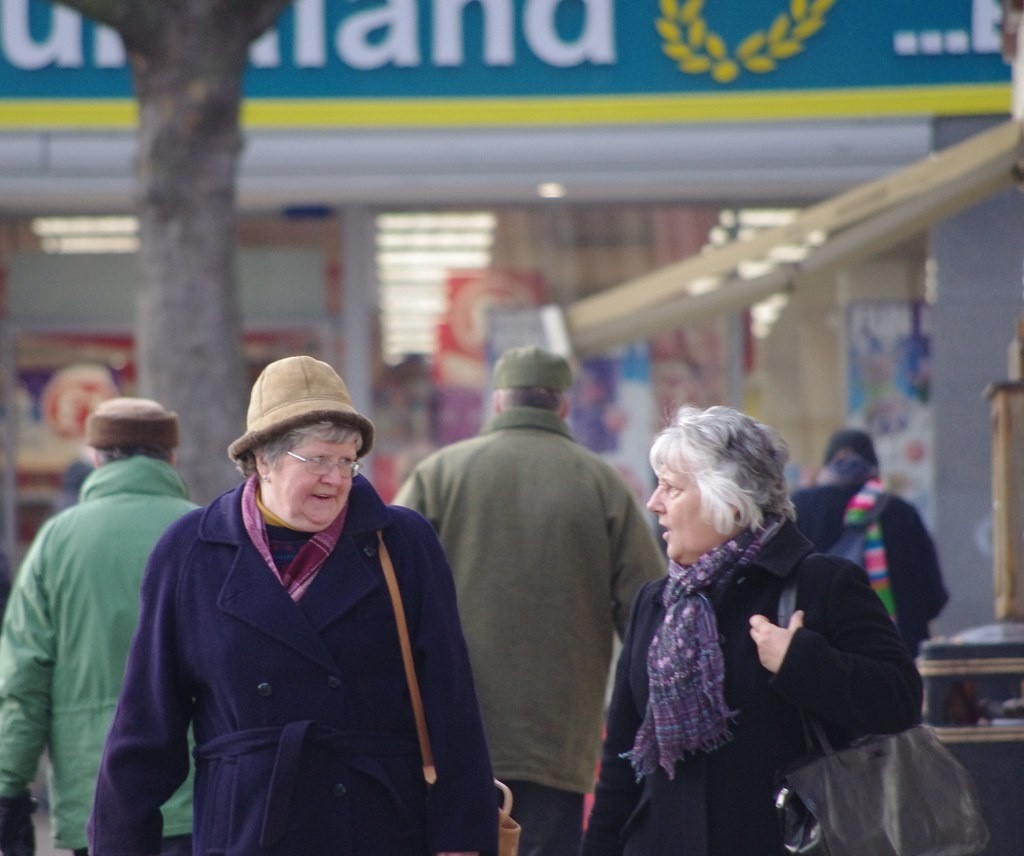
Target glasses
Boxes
[287,451,361,479]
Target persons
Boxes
[1,394,207,856]
[580,404,926,856]
[788,429,949,658]
[391,346,667,856]
[89,356,501,856]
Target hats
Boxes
[823,431,878,465]
[493,345,572,392]
[85,398,179,450]
[227,355,375,462]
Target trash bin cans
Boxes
[912,641,1024,856]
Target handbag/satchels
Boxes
[778,573,989,856]
[495,779,521,856]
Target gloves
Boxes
[0,788,39,856]
